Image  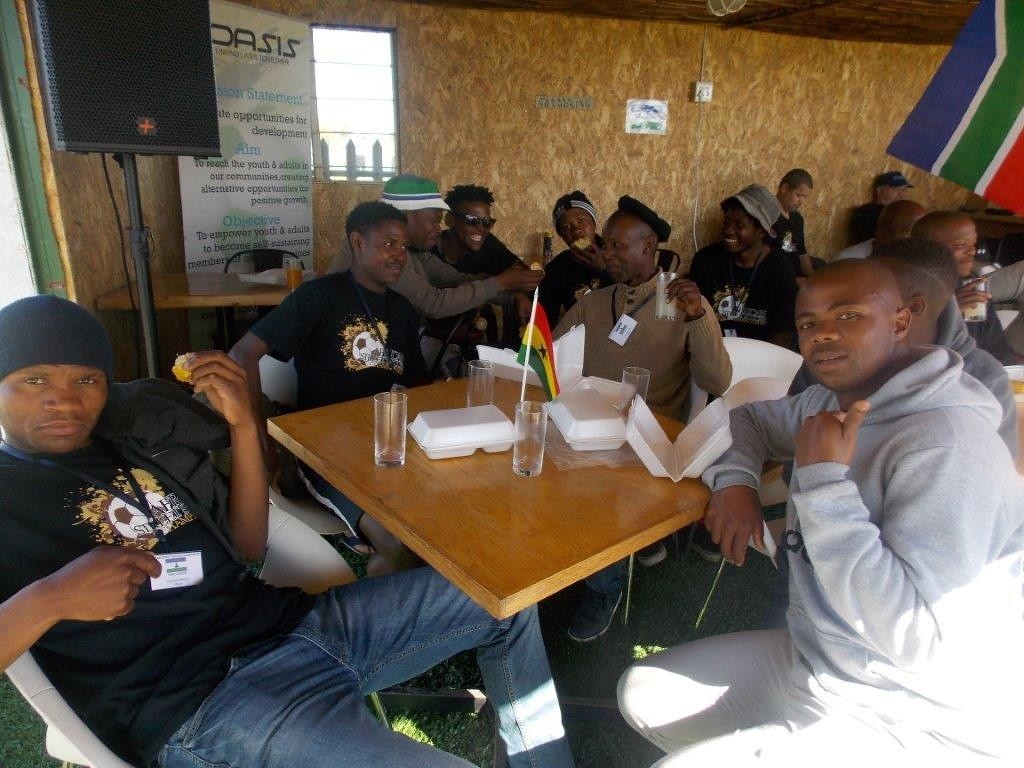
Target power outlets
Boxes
[695,81,714,102]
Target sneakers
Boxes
[567,587,622,641]
[637,541,668,566]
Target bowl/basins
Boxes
[236,268,312,286]
[475,344,543,386]
[406,405,524,459]
[1004,365,1024,403]
[551,322,636,412]
[625,393,732,483]
[542,389,627,451]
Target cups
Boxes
[962,277,986,322]
[466,360,496,407]
[620,367,650,418]
[285,259,303,293]
[655,272,681,320]
[373,391,407,466]
[512,401,548,478]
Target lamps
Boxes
[705,0,747,17]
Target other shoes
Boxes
[691,522,722,560]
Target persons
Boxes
[0,295,576,768]
[226,166,1024,641]
[615,257,1024,768]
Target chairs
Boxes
[687,337,805,425]
[0,493,359,768]
[215,247,308,354]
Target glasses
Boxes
[451,212,497,230]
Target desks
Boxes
[266,366,784,768]
[98,272,294,352]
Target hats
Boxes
[618,194,671,243]
[721,182,780,239]
[380,173,452,210]
[874,170,914,189]
[553,190,598,228]
[0,294,113,398]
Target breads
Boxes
[530,262,545,274]
[171,352,193,382]
[571,238,590,249]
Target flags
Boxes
[887,0,1024,221]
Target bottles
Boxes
[543,231,553,256]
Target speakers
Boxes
[30,0,222,157]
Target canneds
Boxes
[543,231,553,256]
